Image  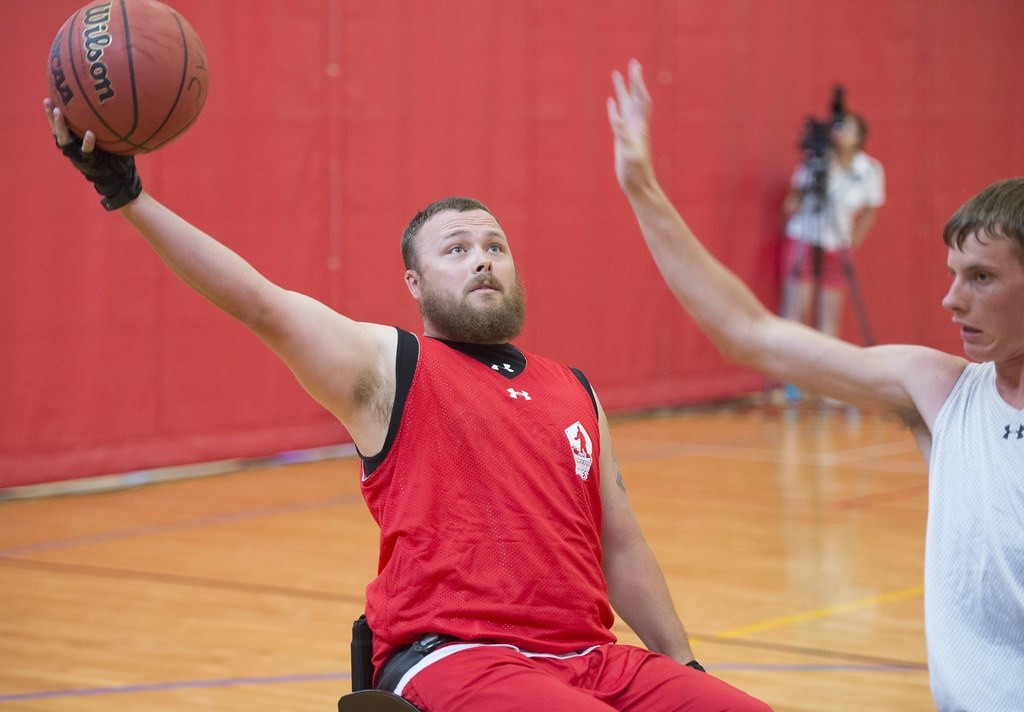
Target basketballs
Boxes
[45,0,209,159]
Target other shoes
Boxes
[786,381,799,399]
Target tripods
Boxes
[785,140,876,349]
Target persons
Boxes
[770,110,887,406]
[42,94,775,712]
[606,58,1023,712]
